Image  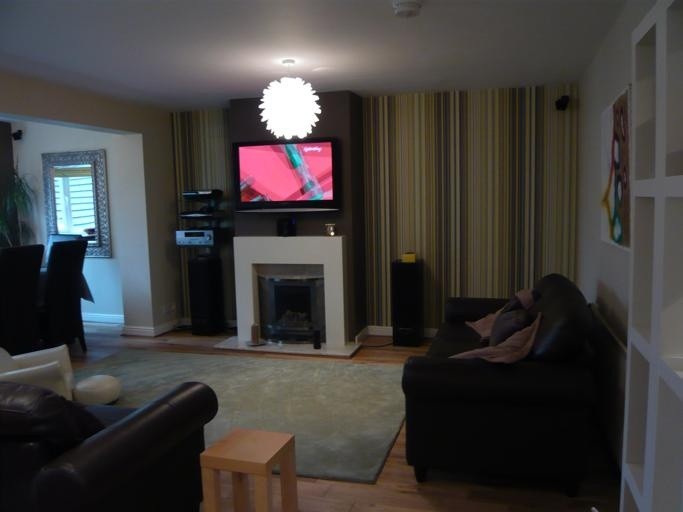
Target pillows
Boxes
[447,293,543,361]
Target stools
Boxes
[198,426,298,511]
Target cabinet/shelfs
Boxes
[619,1,682,510]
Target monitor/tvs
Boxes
[232,137,343,236]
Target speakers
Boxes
[390,259,424,347]
[188,253,226,335]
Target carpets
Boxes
[72,347,406,484]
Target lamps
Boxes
[257,57,322,140]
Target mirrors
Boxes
[49,160,100,249]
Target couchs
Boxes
[399,272,618,497]
